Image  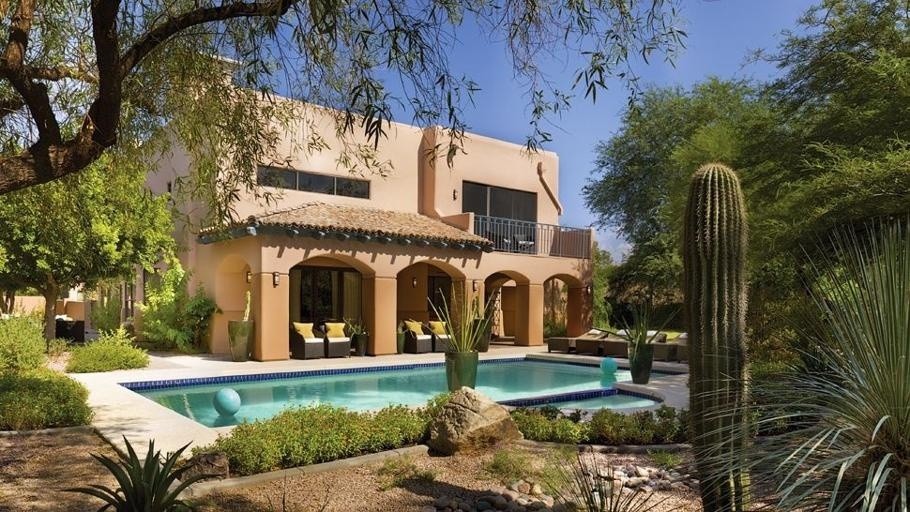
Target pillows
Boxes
[293,321,346,338]
[405,320,447,335]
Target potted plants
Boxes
[397,318,408,354]
[591,292,680,385]
[343,317,369,357]
[409,282,497,393]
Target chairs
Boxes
[289,321,353,360]
[486,229,535,253]
[548,327,689,363]
[404,320,454,354]
